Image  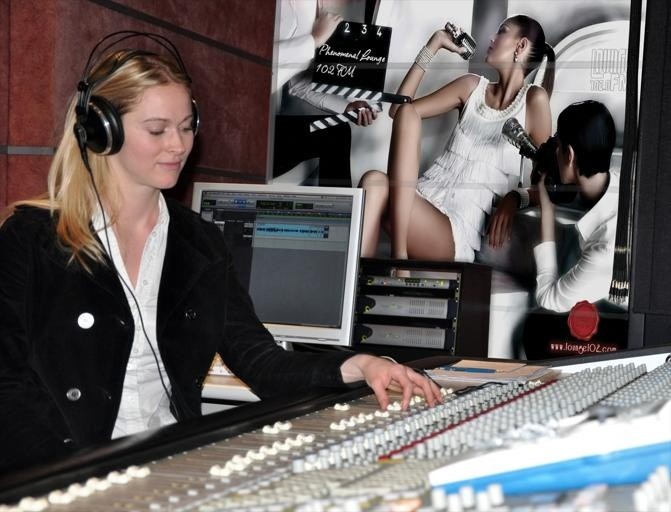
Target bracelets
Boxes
[514,186,532,210]
[414,45,437,75]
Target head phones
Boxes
[74,30,202,171]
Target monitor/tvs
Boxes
[190,180,365,379]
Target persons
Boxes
[272,0,380,187]
[0,46,446,486]
[354,13,560,280]
[520,96,635,362]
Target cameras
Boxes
[520,135,563,203]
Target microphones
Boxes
[445,22,477,60]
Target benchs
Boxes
[473,203,587,359]
